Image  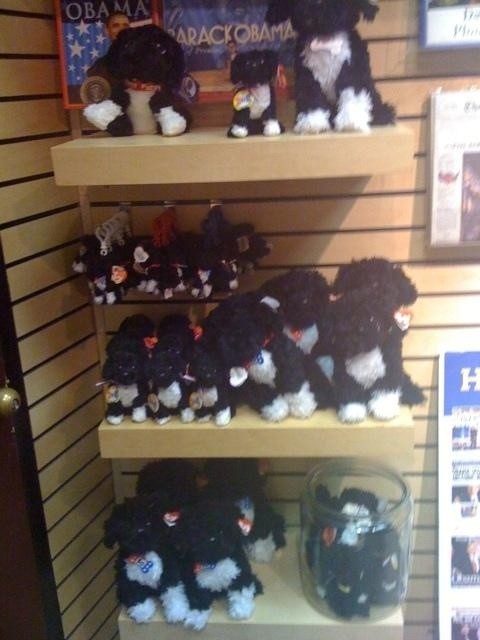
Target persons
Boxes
[451,540,480,574]
[223,41,239,65]
[452,484,480,503]
[462,164,480,239]
[105,12,130,42]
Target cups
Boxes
[297,461,415,623]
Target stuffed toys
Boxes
[263,1,397,135]
[71,205,270,308]
[103,456,286,631]
[225,48,286,138]
[70,1,428,633]
[306,484,407,619]
[99,257,426,426]
[83,23,194,137]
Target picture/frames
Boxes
[416,0,479,53]
[428,90,480,261]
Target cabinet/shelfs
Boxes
[52,122,415,640]
